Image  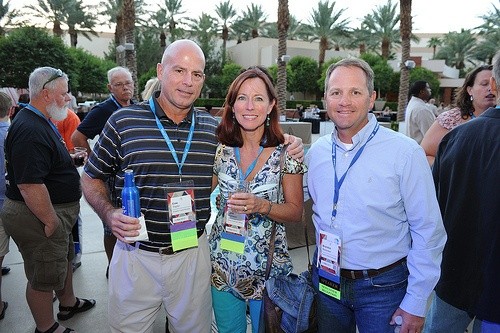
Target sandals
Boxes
[57,297,96,320]
[35,322,78,333]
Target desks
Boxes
[277,109,328,145]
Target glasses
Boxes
[42,69,63,89]
[111,82,133,89]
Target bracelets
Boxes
[265,200,272,216]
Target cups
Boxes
[227,180,248,212]
[74,146,87,166]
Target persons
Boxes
[141,78,161,102]
[210,68,305,333]
[423,50,500,333]
[50,105,81,272]
[419,64,497,167]
[404,81,438,142]
[78,38,303,333]
[72,66,136,276]
[11,92,30,121]
[216,57,447,333]
[65,92,78,113]
[383,106,392,117]
[425,97,438,118]
[1,66,96,333]
[312,105,321,116]
[0,91,17,320]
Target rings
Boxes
[243,206,248,211]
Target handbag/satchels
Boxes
[262,267,318,333]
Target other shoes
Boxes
[2,267,10,275]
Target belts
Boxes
[340,260,403,278]
[130,243,174,256]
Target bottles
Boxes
[121,169,141,218]
[395,316,403,326]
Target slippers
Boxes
[0,301,8,319]
